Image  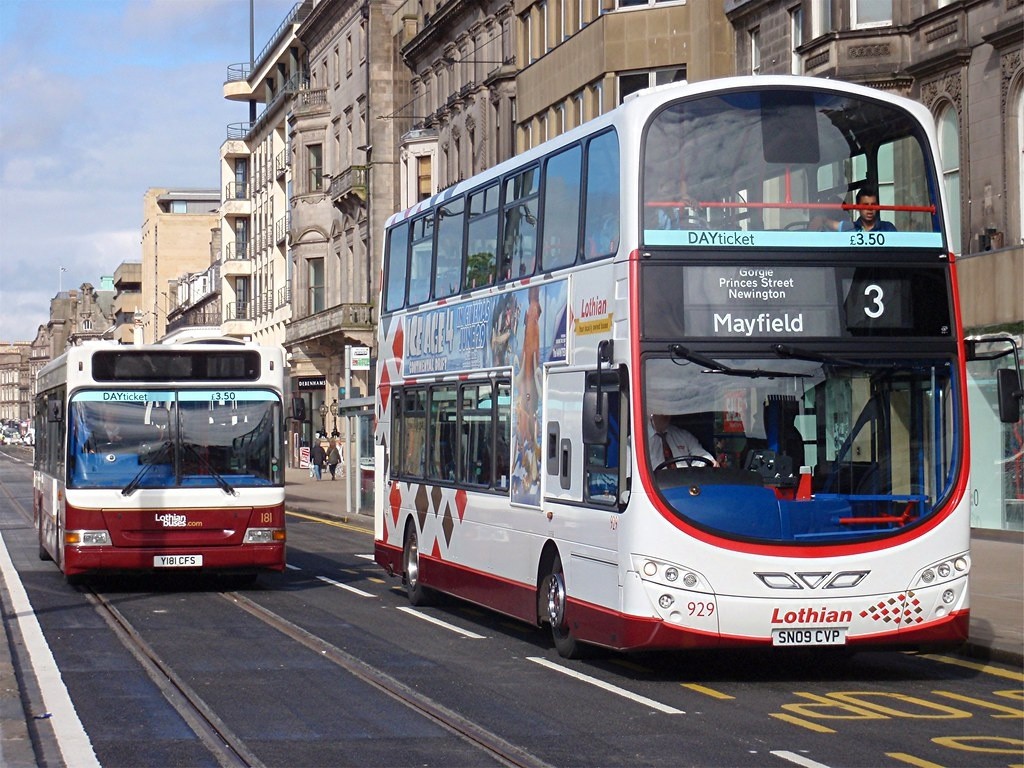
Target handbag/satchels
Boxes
[309,463,314,478]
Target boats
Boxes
[34,337,306,586]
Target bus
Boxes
[374,74,1024,659]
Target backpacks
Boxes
[329,452,338,465]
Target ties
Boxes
[655,432,676,468]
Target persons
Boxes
[644,414,719,470]
[84,408,139,453]
[326,440,341,480]
[810,185,897,232]
[309,440,326,481]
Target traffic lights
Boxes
[338,387,346,417]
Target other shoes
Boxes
[316,478,320,481]
[332,474,337,481]
